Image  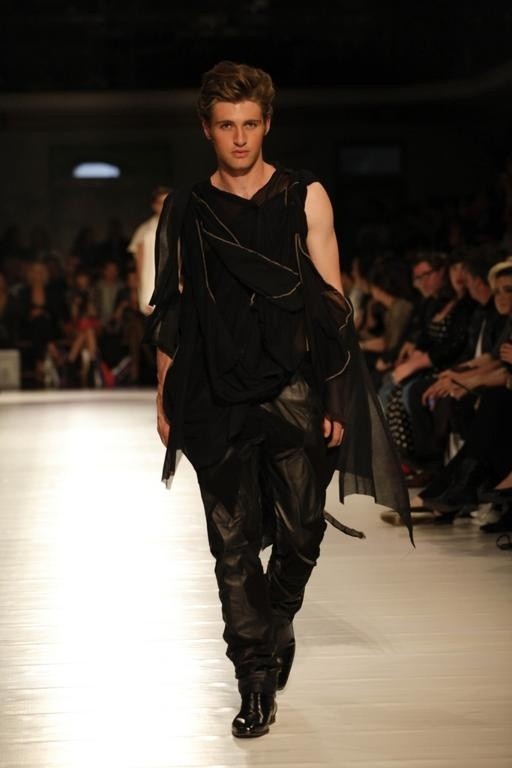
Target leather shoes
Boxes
[273,637,294,692]
[232,693,278,738]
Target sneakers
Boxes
[381,507,435,525]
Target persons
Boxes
[153,62,414,739]
[0,190,512,554]
